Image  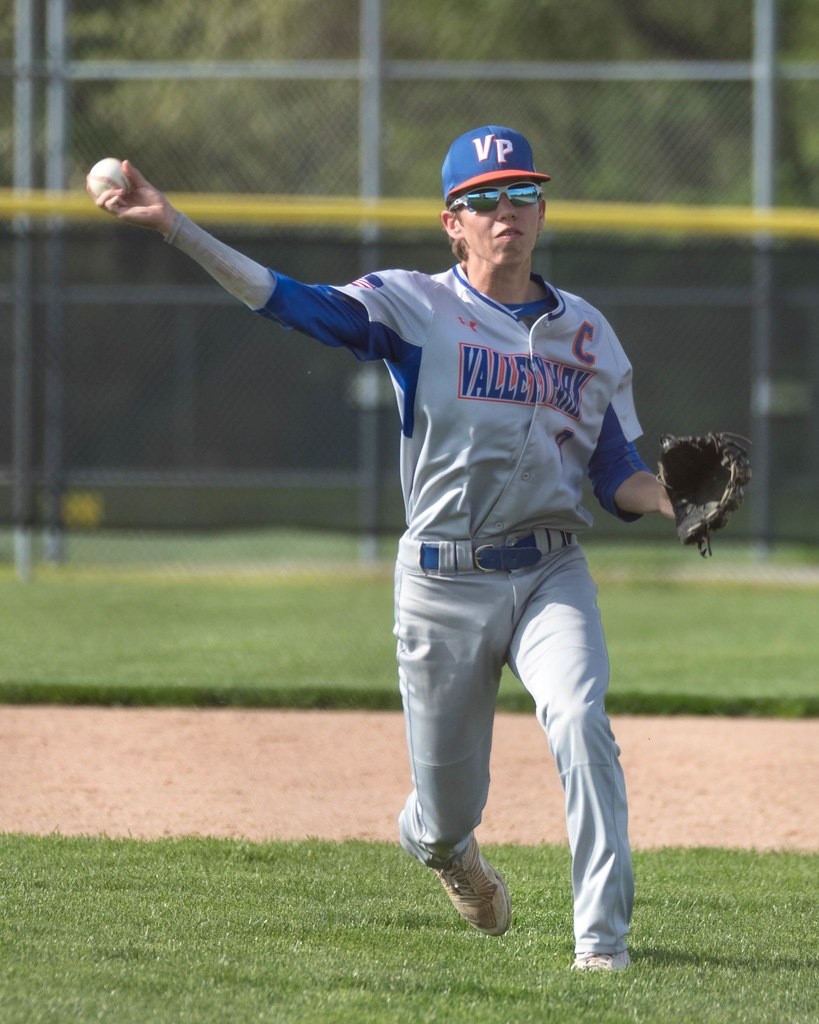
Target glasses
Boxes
[449,181,544,211]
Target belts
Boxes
[420,529,571,572]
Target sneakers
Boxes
[433,829,512,937]
[571,950,630,972]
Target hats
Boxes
[442,125,552,206]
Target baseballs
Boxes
[89,157,132,201]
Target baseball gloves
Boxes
[655,431,753,558]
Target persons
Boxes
[84,125,753,974]
